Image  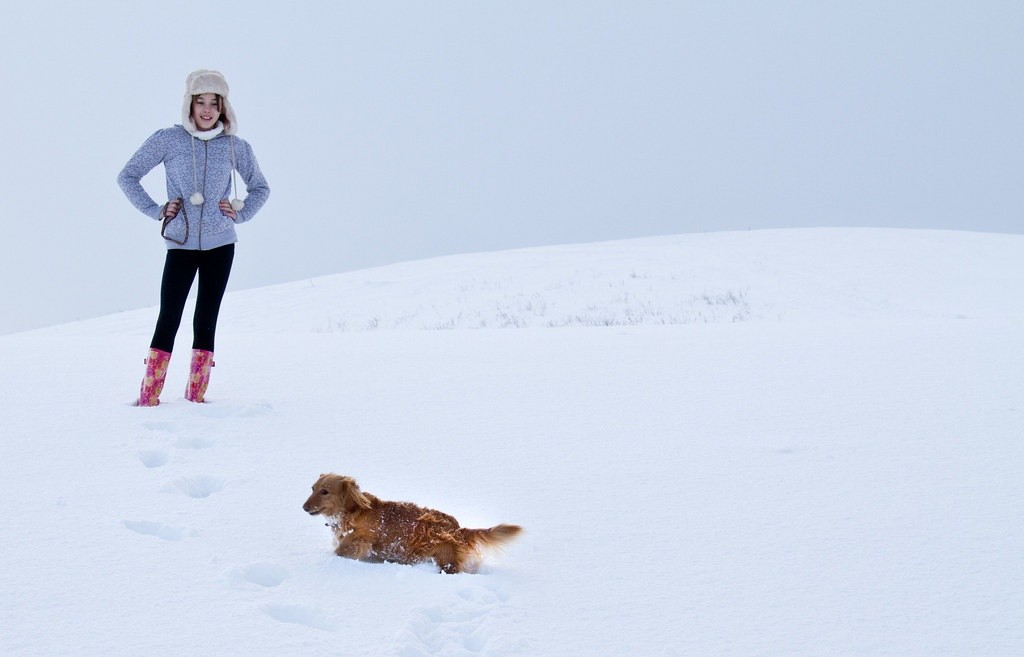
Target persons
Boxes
[117,69,270,406]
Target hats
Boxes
[182,70,244,211]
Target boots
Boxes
[137,348,171,408]
[183,348,215,403]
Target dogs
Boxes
[302,473,525,575]
